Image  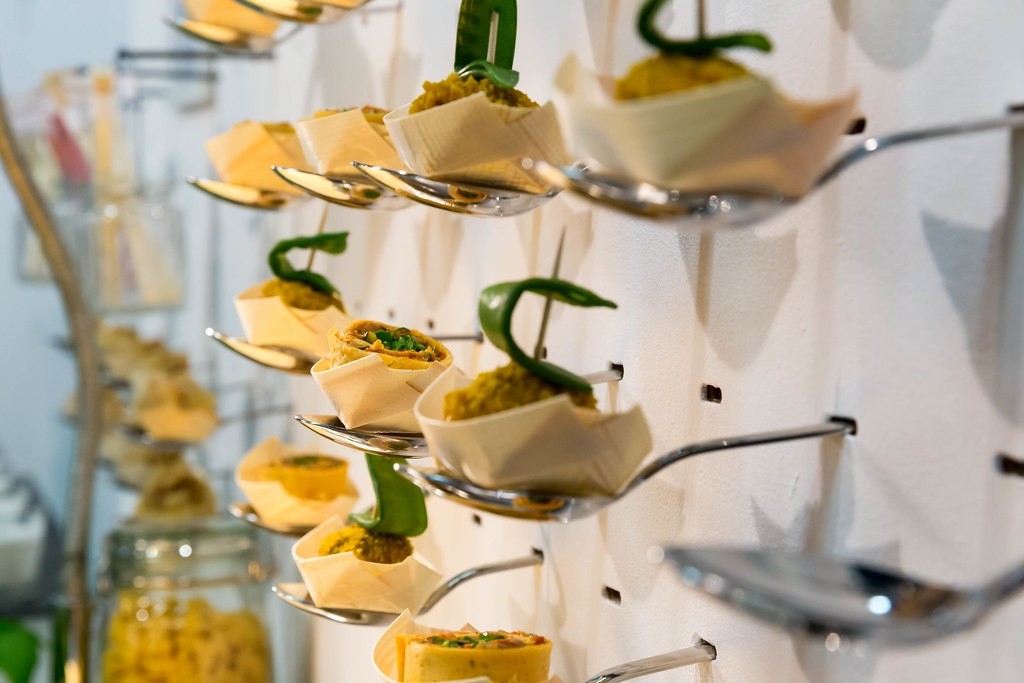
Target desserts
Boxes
[66,0,863,683]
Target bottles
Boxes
[94,521,275,683]
[16,173,183,313]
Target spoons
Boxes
[272,166,414,211]
[205,329,482,375]
[659,542,1024,646]
[272,554,543,626]
[163,17,305,54]
[296,371,623,459]
[239,0,372,27]
[522,110,1024,229]
[227,488,430,538]
[185,176,286,210]
[352,161,590,218]
[394,419,854,524]
